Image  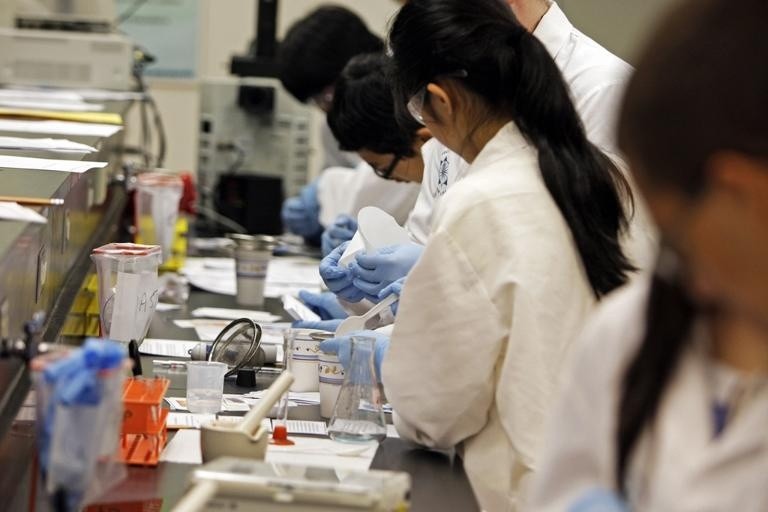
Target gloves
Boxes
[318,239,364,304]
[298,288,350,321]
[291,319,344,332]
[348,243,428,306]
[378,276,407,318]
[320,213,358,260]
[280,175,322,241]
[319,329,392,387]
[566,489,632,512]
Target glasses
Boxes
[405,69,469,127]
[373,153,402,181]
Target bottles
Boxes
[325,337,389,444]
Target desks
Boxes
[0,237,480,512]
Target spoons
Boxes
[332,293,397,335]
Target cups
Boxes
[133,175,182,265]
[186,361,228,413]
[288,328,340,419]
[233,249,272,306]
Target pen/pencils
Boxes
[0,196,65,206]
[129,340,162,454]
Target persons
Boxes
[276,1,653,512]
[530,236,768,511]
[616,1,768,312]
